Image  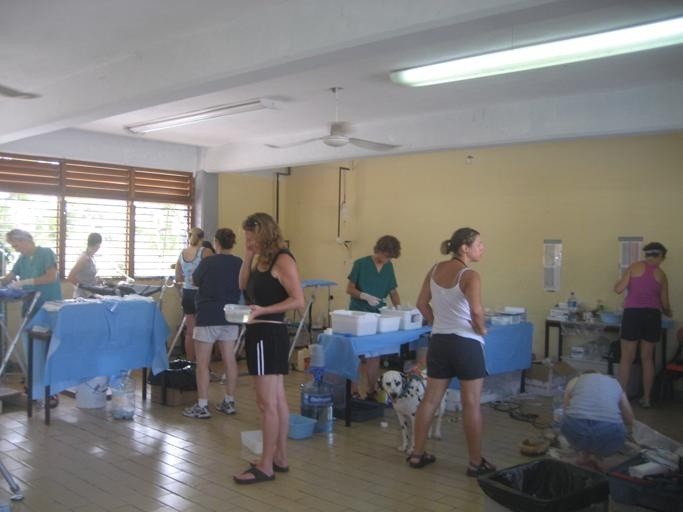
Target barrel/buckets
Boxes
[110,369,135,419]
[74,376,108,409]
[299,367,336,433]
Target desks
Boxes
[293,279,338,320]
[545,321,667,371]
[24,328,168,426]
[318,322,532,428]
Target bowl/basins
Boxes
[518,436,551,456]
[224,304,252,323]
[599,310,624,324]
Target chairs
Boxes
[657,326,683,409]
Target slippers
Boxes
[233,466,275,484]
[249,453,289,474]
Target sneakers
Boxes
[216,399,236,414]
[180,403,210,419]
[47,393,59,408]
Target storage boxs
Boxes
[607,453,683,512]
[148,358,209,408]
[524,361,578,397]
[380,304,422,331]
[376,313,402,332]
[288,414,317,438]
[330,309,380,335]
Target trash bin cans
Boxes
[148,359,198,407]
[476,458,610,512]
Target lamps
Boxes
[125,97,282,136]
[391,16,683,88]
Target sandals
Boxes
[466,456,495,478]
[408,451,435,469]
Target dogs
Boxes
[375,369,450,455]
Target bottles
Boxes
[551,384,566,423]
[568,290,577,323]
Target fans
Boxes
[265,88,402,151]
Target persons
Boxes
[344,234,403,404]
[181,228,243,419]
[68,232,102,299]
[175,228,213,363]
[559,369,634,467]
[614,241,673,408]
[232,212,305,485]
[0,228,61,408]
[406,227,497,477]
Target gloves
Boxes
[359,292,381,308]
[8,278,34,292]
